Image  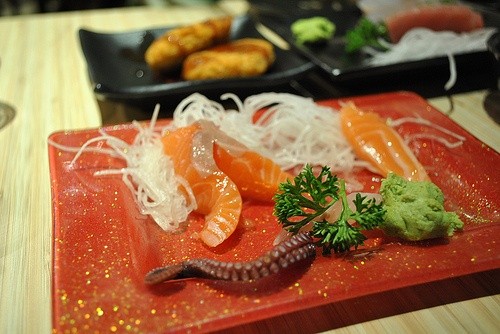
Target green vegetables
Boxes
[339,20,390,54]
[272,163,388,256]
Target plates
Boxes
[76,5,316,112]
[46,90,500,334]
[257,5,500,95]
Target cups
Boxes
[486,30,500,104]
